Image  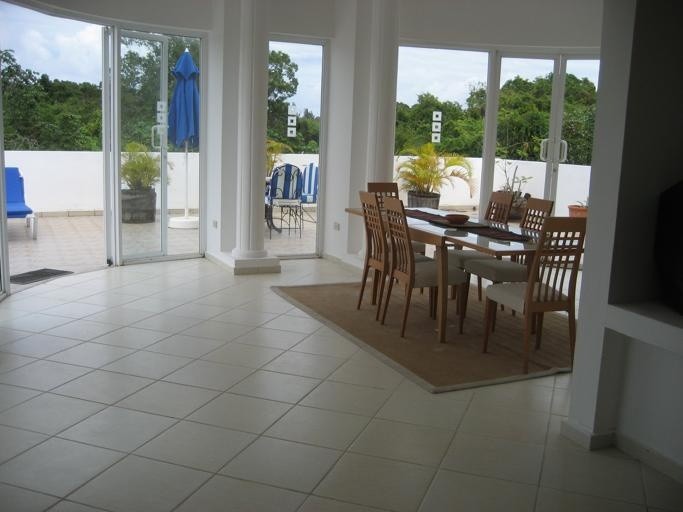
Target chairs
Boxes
[381,196,468,344]
[264,163,302,238]
[366,182,425,296]
[358,190,434,320]
[464,191,515,302]
[478,197,554,318]
[482,216,586,374]
[3,167,37,239]
[299,163,318,224]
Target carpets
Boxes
[269,275,576,393]
[9,267,72,287]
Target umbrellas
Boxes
[166,42,201,230]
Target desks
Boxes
[263,177,281,234]
[345,205,585,344]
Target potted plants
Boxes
[393,142,478,210]
[495,158,535,220]
[115,143,173,223]
